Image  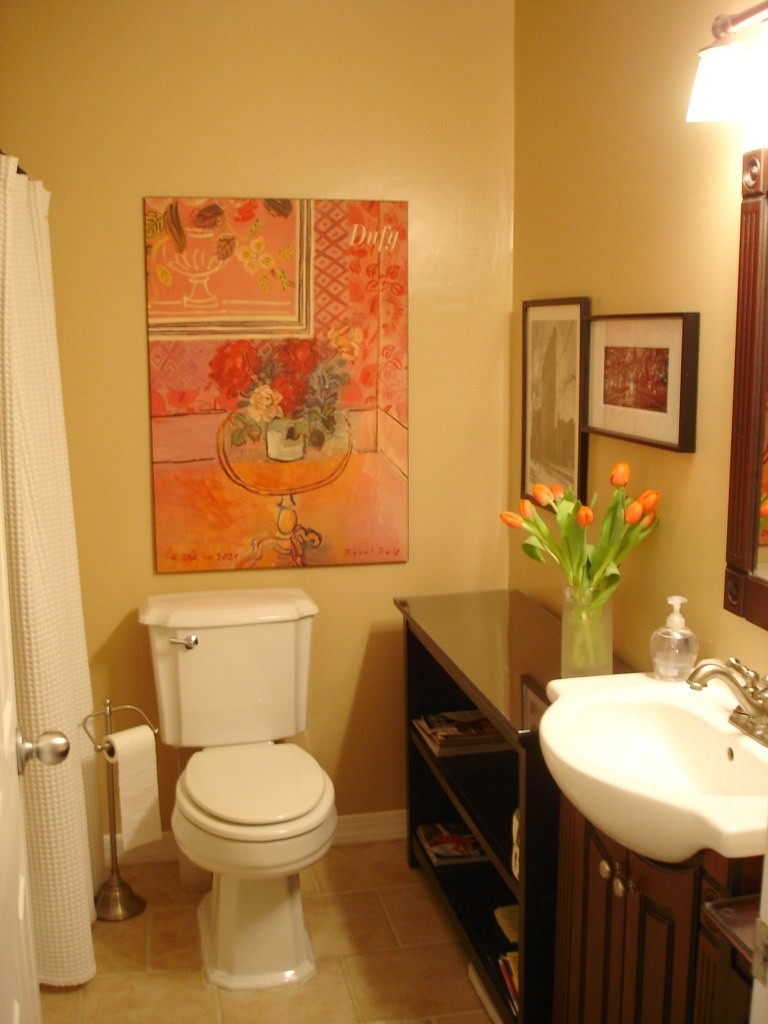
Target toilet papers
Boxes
[102,723,162,766]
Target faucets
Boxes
[684,658,765,723]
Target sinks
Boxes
[538,672,767,867]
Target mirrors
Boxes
[723,146,768,633]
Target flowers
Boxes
[758,443,768,546]
[499,460,660,670]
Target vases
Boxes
[560,589,613,678]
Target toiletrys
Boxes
[649,594,699,683]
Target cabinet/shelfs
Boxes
[701,893,760,1024]
[554,786,762,1024]
[394,590,639,1023]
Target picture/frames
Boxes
[521,296,592,514]
[579,312,701,452]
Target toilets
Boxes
[137,588,339,989]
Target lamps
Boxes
[685,0,768,125]
